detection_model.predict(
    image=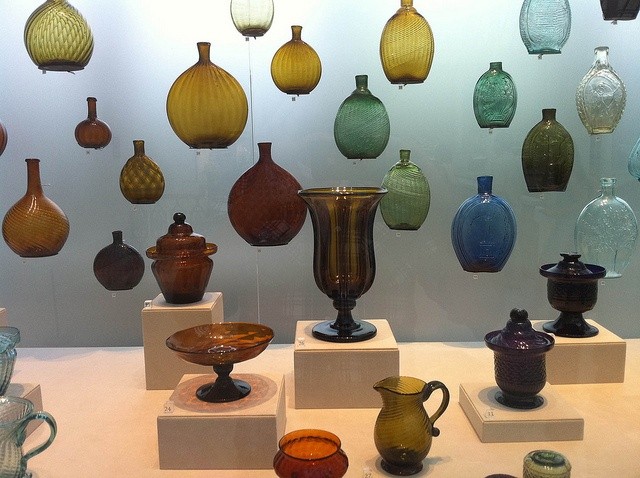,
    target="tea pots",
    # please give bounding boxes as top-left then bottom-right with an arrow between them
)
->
373,376 -> 450,476
